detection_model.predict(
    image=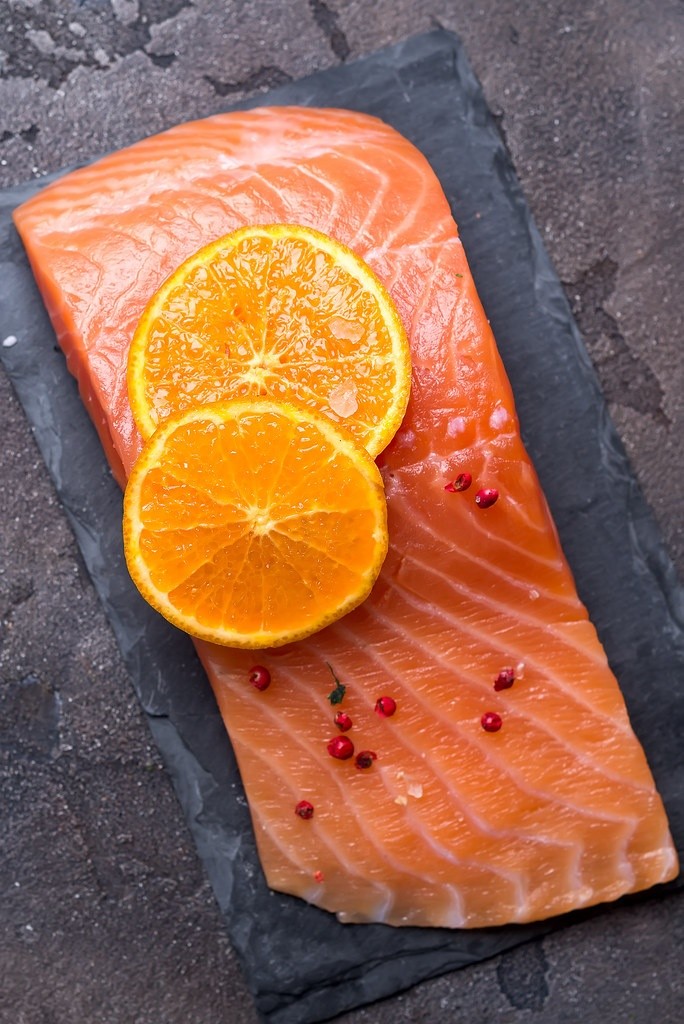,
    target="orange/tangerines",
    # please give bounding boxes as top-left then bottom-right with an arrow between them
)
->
128,222 -> 410,464
120,398 -> 389,650
